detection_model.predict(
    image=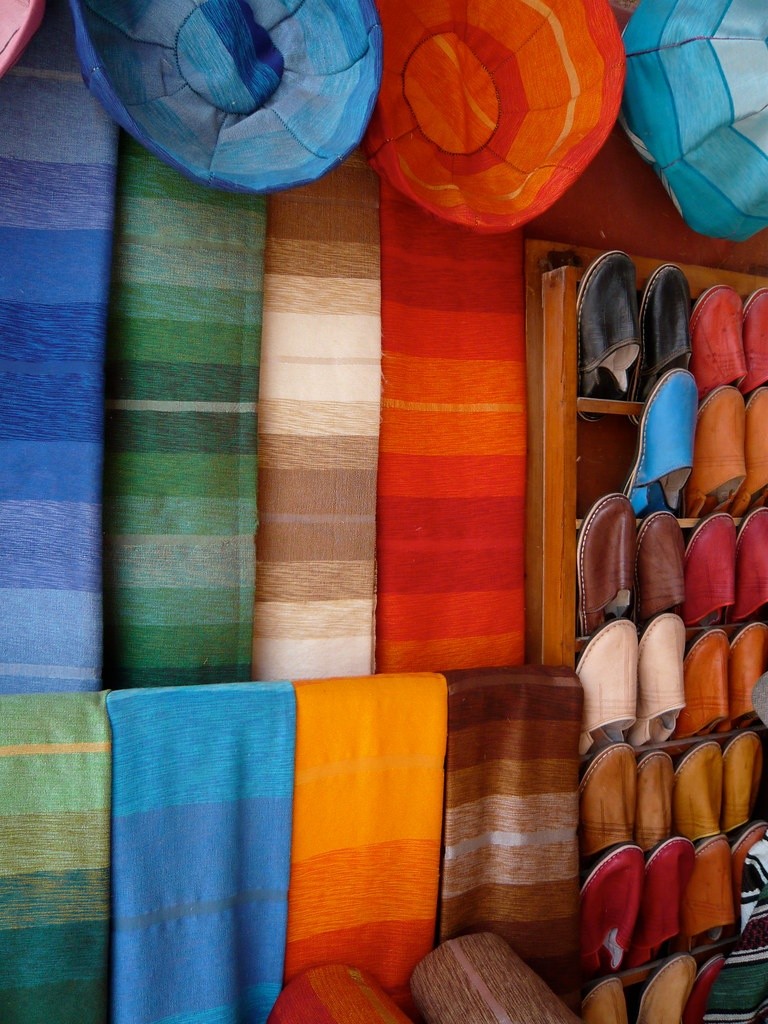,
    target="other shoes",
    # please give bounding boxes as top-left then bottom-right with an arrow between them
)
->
577,245 -> 768,1024
